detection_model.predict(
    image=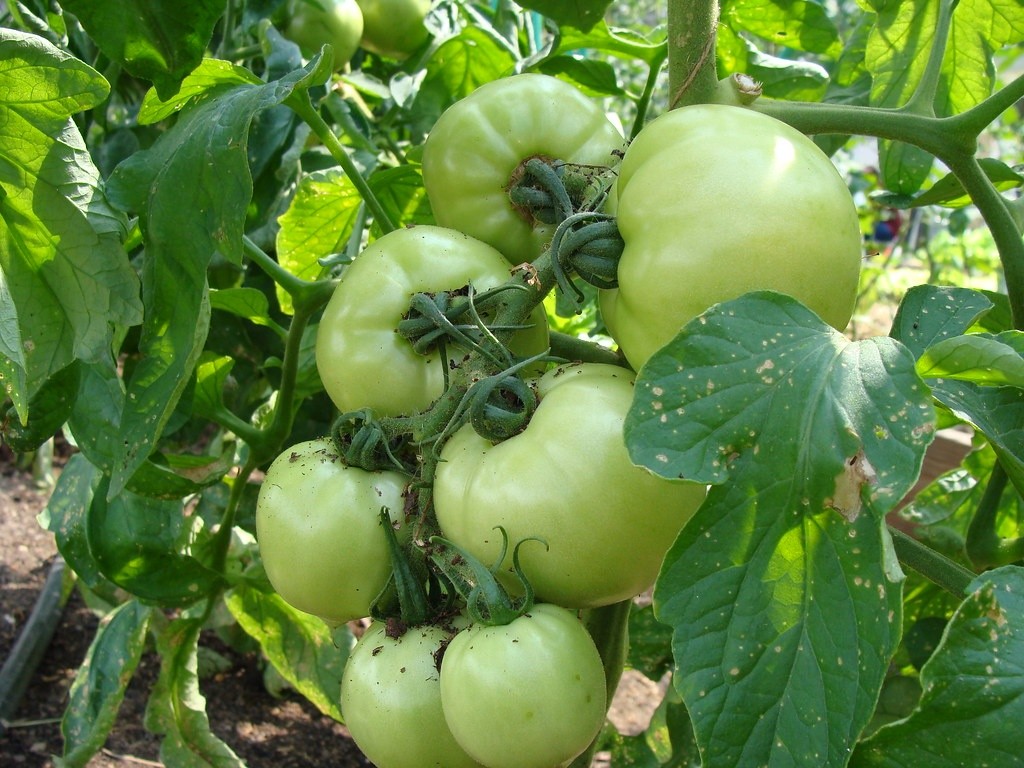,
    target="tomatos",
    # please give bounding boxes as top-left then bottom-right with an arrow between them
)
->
357,1 -> 434,62
254,74 -> 861,767
283,0 -> 362,73
